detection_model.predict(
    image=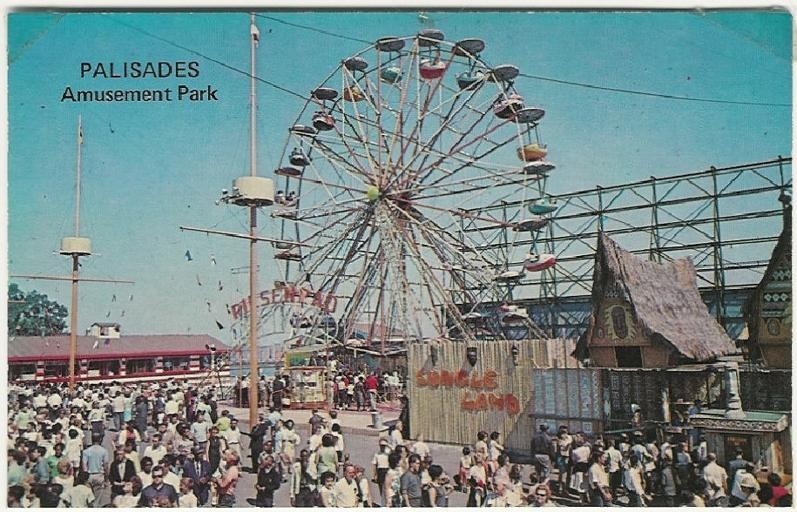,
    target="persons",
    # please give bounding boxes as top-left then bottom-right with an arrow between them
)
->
277,190 -> 296,202
291,146 -> 298,154
7,350 -> 792,507
